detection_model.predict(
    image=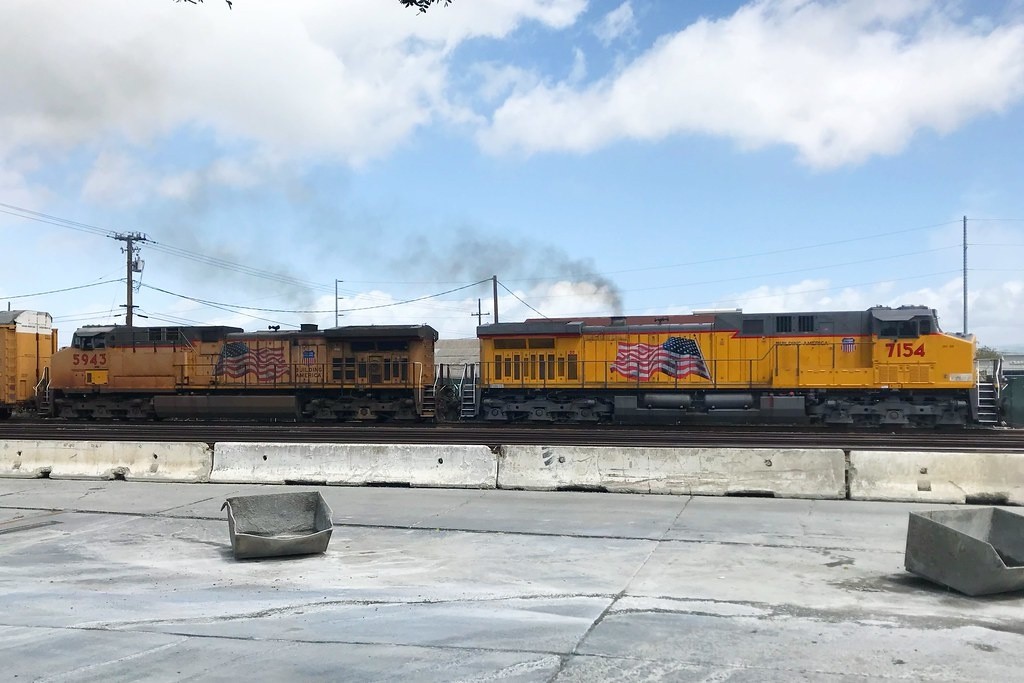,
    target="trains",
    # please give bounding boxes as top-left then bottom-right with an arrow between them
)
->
0,302 -> 1023,431
